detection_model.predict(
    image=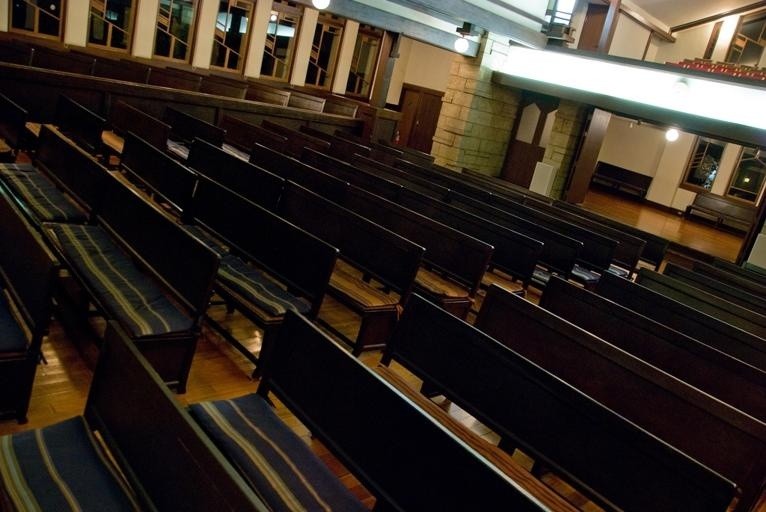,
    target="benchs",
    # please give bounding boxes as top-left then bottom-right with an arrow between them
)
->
2,189 -> 60,425
118,133 -> 340,380
683,192 -> 760,238
3,321 -> 267,511
2,124 -> 223,393
0,36 -> 403,167
185,133 -> 675,376
469,247 -> 764,508
368,291 -> 742,511
192,310 -> 551,512
